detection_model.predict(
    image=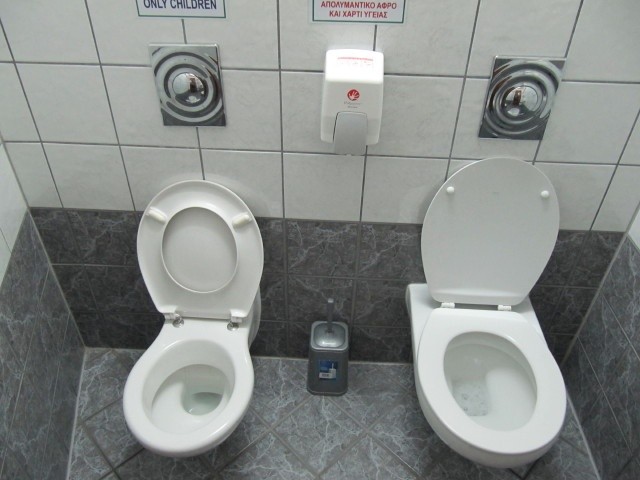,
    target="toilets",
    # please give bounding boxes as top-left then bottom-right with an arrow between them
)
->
121,179 -> 264,457
405,156 -> 566,468
